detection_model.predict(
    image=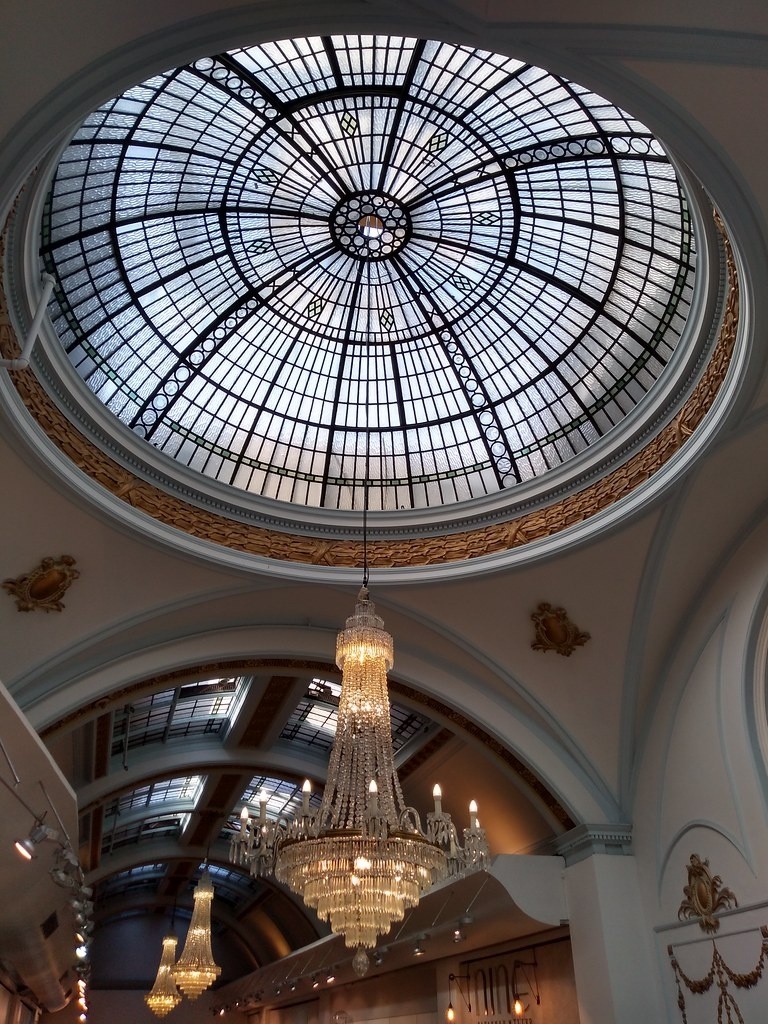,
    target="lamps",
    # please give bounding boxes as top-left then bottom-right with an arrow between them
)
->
71,901 -> 93,924
75,920 -> 94,943
13,820 -> 56,859
48,849 -> 80,889
371,953 -> 386,967
76,938 -> 93,959
310,976 -> 319,988
169,839 -> 222,1002
410,944 -> 425,959
142,933 -> 183,1019
324,971 -> 335,984
448,924 -> 468,943
228,239 -> 492,978
66,882 -> 93,912
